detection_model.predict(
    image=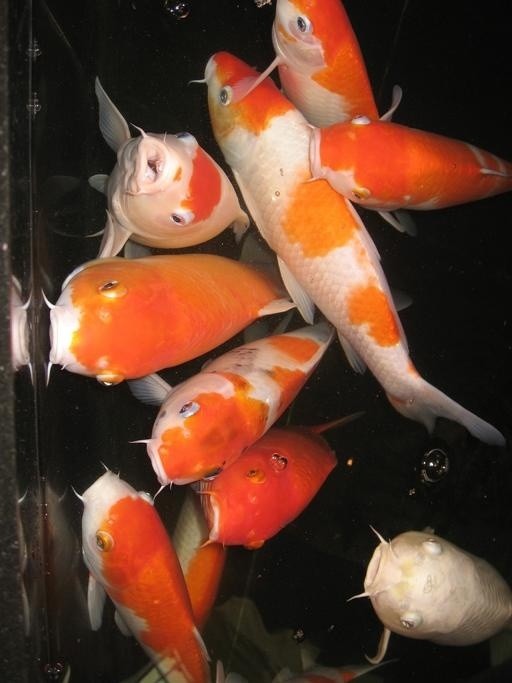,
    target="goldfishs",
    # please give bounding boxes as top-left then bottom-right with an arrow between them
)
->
171,405 -> 371,635
185,48 -> 512,449
